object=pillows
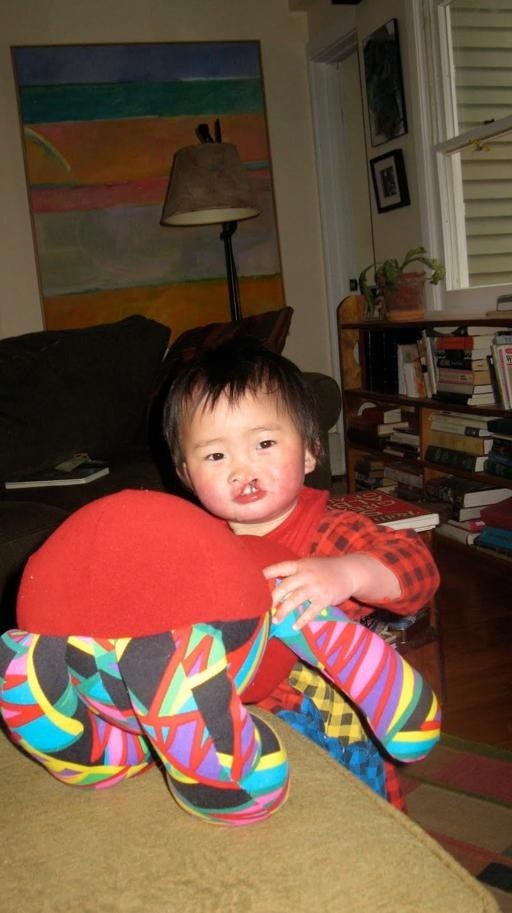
[143,306,293,442]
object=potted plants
[358,247,445,322]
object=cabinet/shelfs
[336,295,512,570]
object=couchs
[0,315,342,630]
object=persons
[161,333,442,819]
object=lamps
[163,119,260,321]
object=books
[3,465,111,490]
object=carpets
[399,735,510,913]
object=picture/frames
[362,17,411,214]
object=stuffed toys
[0,485,442,826]
